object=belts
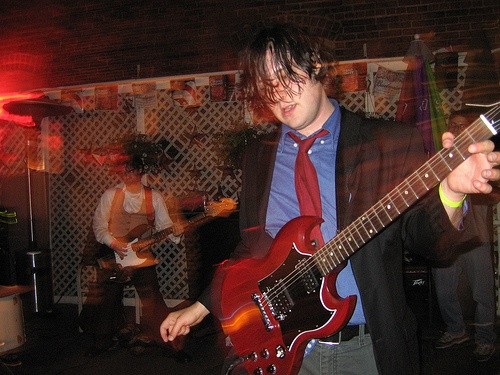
[315,323,369,345]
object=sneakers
[473,343,497,362]
[434,330,471,351]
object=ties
[288,129,329,251]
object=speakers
[403,264,436,324]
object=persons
[434,110,500,361]
[92,139,186,344]
[160,22,500,375]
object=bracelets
[439,182,467,208]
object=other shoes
[85,343,108,359]
[165,349,194,362]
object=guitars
[208,101,500,375]
[86,193,237,277]
